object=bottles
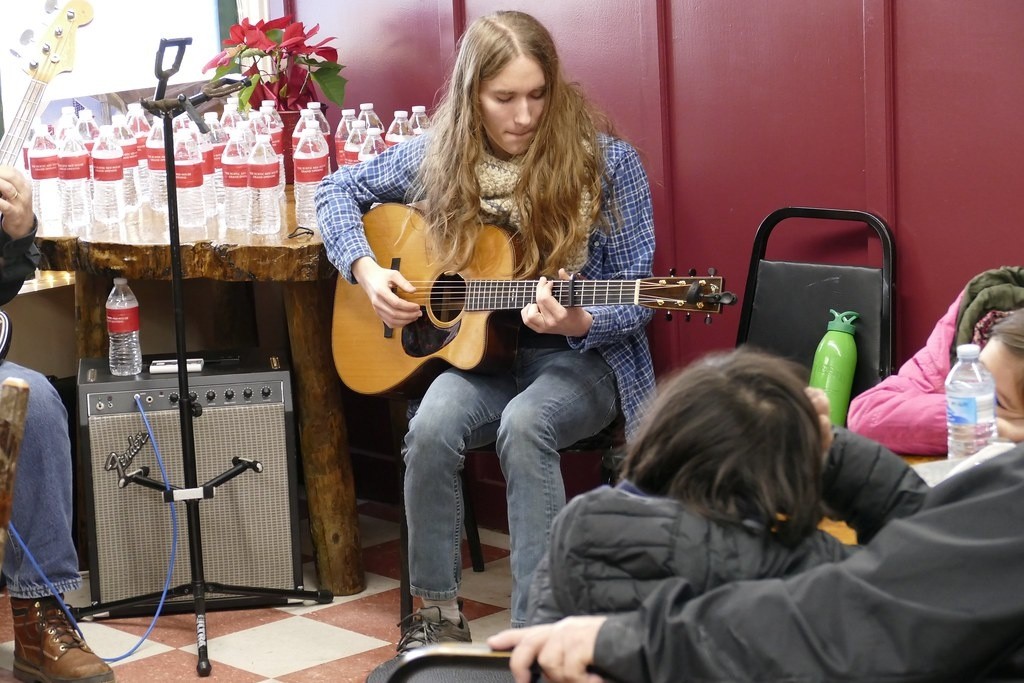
[945,344,997,474]
[19,98,434,236]
[105,278,142,377]
[809,309,860,428]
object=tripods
[63,37,336,674]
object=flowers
[200,14,349,109]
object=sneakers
[396,600,472,652]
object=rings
[8,193,18,200]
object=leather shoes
[10,593,114,683]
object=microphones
[231,456,264,473]
[119,466,149,489]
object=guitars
[324,192,746,401]
[0,0,101,374]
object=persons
[0,165,114,683]
[523,349,929,628]
[849,264,1023,454]
[315,11,654,656]
[488,444,1024,683]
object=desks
[22,183,369,596]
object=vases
[277,100,329,184]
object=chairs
[399,416,626,634]
[735,207,896,397]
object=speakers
[73,349,305,606]
